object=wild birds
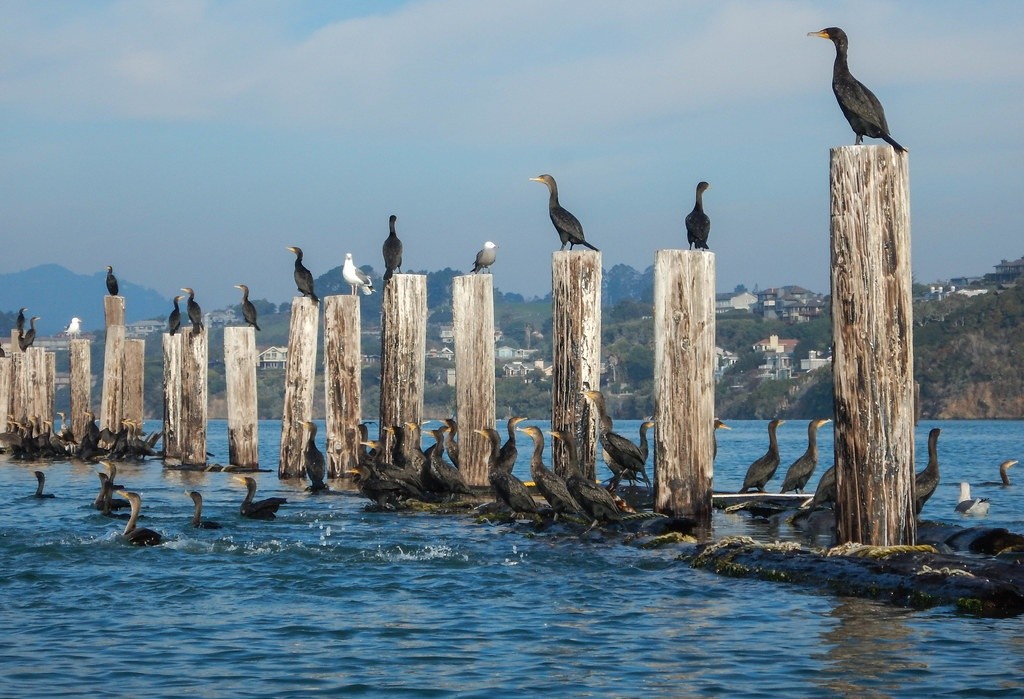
[180,288,204,330]
[33,471,54,498]
[529,175,598,251]
[106,265,118,296]
[299,418,475,511]
[685,182,711,250]
[382,215,403,280]
[475,392,654,538]
[65,317,81,340]
[914,427,1018,519]
[0,411,164,463]
[342,253,376,296]
[234,285,260,331]
[470,241,500,274]
[807,27,906,153]
[16,307,40,353]
[287,247,319,302]
[94,460,288,549]
[713,416,836,510]
[168,295,185,336]
